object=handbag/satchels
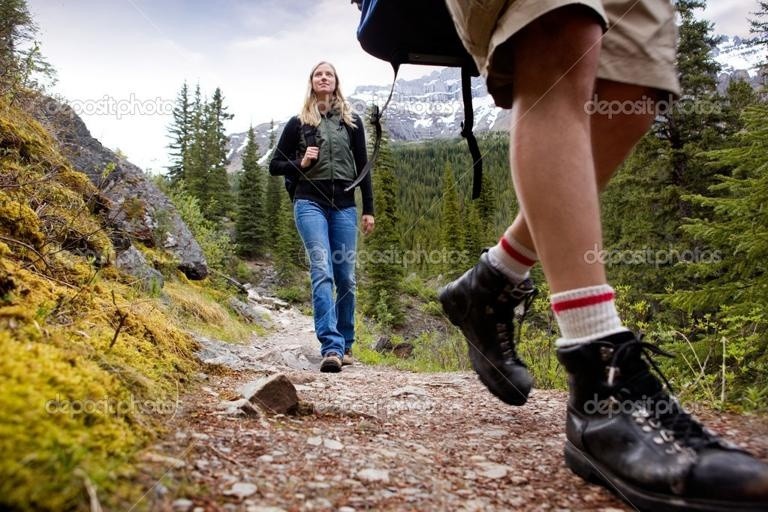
[285,114,316,202]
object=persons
[438,0,768,512]
[269,61,376,372]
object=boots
[557,331,768,512]
[438,252,531,405]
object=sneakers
[342,348,353,364]
[320,351,342,373]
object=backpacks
[351,0,480,76]
